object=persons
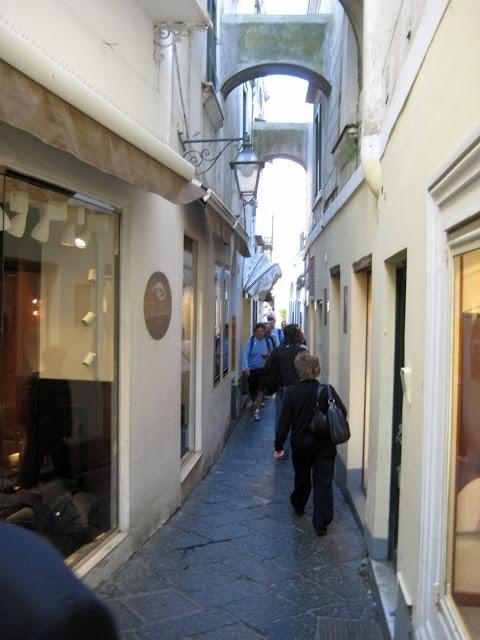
[272,352,348,537]
[216,324,229,374]
[21,346,75,488]
[181,326,191,426]
[242,316,310,460]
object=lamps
[179,130,265,204]
[0,190,97,369]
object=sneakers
[294,507,304,516]
[315,528,326,536]
[254,412,260,420]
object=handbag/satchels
[309,400,350,444]
[257,362,277,394]
